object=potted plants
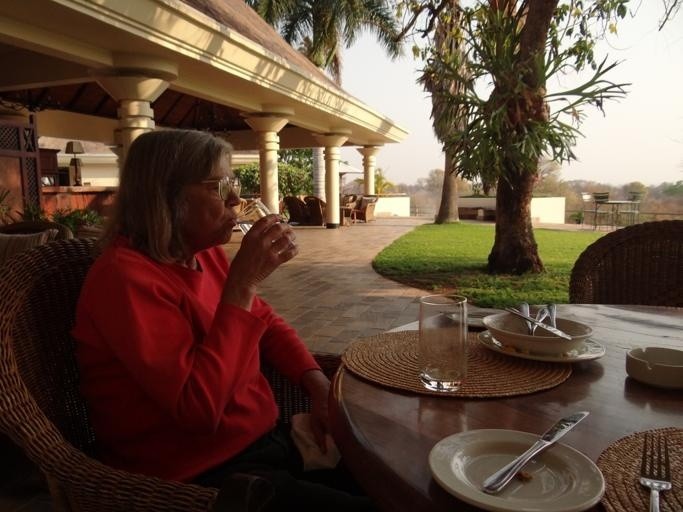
[625,184,641,200]
[571,205,584,224]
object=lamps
[66,141,84,187]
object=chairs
[569,220,683,304]
[1,238,341,509]
[283,194,379,226]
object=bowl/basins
[482,313,593,352]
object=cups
[419,294,469,392]
[233,197,271,234]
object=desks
[593,200,640,228]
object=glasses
[185,177,243,199]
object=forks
[639,433,673,511]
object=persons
[69,127,379,512]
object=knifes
[484,411,590,494]
[505,305,571,340]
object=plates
[442,308,493,329]
[428,428,606,511]
[477,330,606,364]
